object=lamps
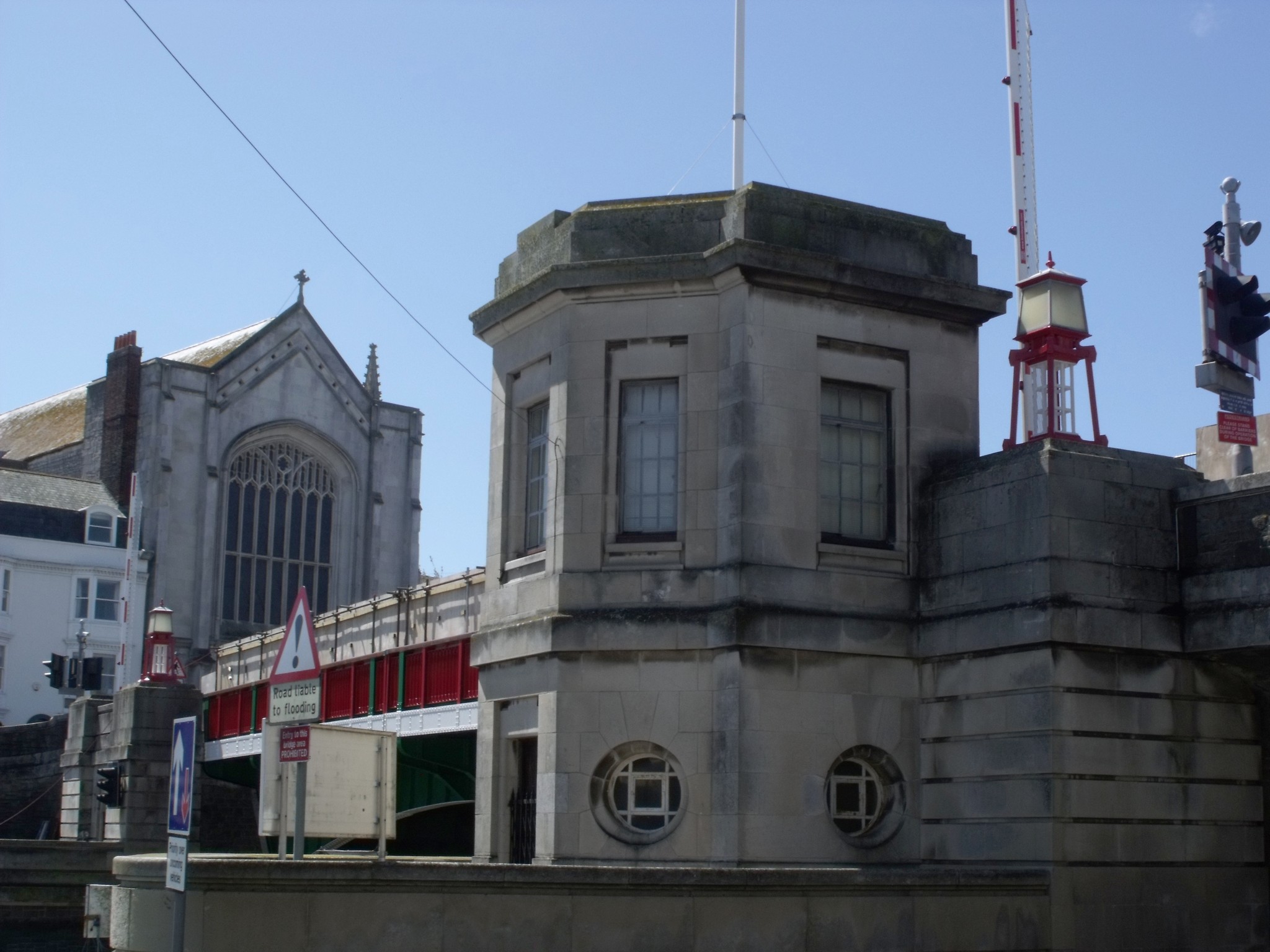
[1001,250,1109,451]
[139,598,178,684]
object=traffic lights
[41,649,61,689]
[83,656,103,691]
[1215,269,1268,358]
[93,763,124,810]
